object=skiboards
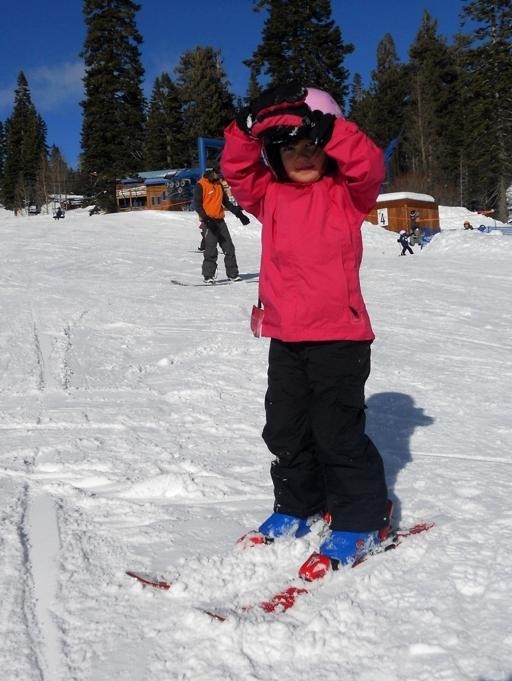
[125,508,435,622]
[172,274,260,287]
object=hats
[213,163,224,178]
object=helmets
[464,220,470,227]
[247,84,345,182]
[399,229,406,235]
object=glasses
[249,83,309,125]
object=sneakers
[297,498,394,582]
[202,276,215,283]
[235,509,323,551]
[228,275,242,281]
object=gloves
[305,106,336,149]
[202,215,221,235]
[235,98,261,140]
[237,211,250,225]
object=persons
[194,163,252,284]
[463,221,474,230]
[409,208,424,249]
[55,207,63,221]
[218,86,393,563]
[396,230,415,256]
